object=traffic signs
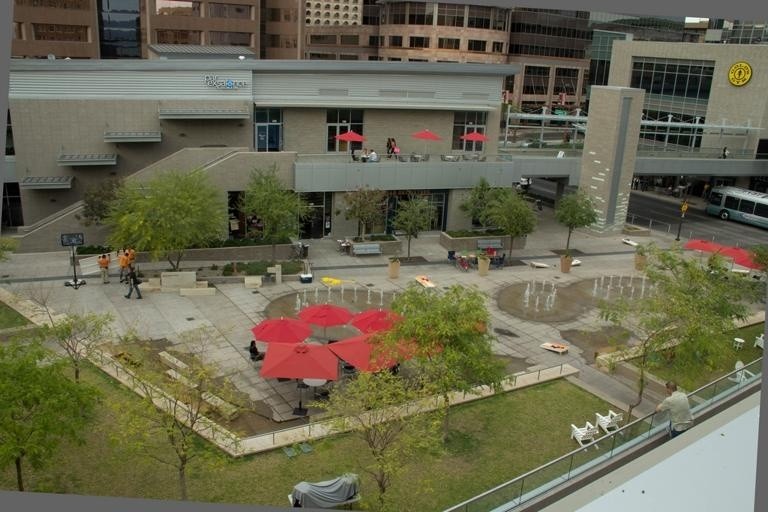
[553,109,566,115]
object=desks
[335,238,351,256]
[286,491,364,512]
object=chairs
[293,363,355,401]
[594,407,627,438]
[246,348,264,369]
[350,151,488,164]
[446,245,507,276]
[569,420,599,452]
[734,360,755,383]
[700,262,762,282]
[753,331,765,350]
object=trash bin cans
[301,243,309,258]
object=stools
[733,336,746,351]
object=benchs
[476,238,505,252]
[352,242,383,258]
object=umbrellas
[734,254,761,272]
[410,130,442,153]
[335,130,366,155]
[682,239,721,263]
[717,247,749,267]
[460,133,490,141]
[251,304,444,411]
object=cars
[570,122,586,133]
[518,177,532,186]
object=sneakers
[124,294,131,299]
[135,296,144,300]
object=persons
[98,246,143,299]
[360,148,369,162]
[369,149,378,162]
[656,380,694,437]
[249,340,265,361]
[723,146,727,158]
[632,176,639,190]
[386,138,392,154]
[388,138,397,159]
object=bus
[702,184,767,230]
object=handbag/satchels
[135,279,142,285]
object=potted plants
[386,251,401,278]
[558,252,574,274]
[633,243,648,272]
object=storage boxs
[299,273,314,283]
